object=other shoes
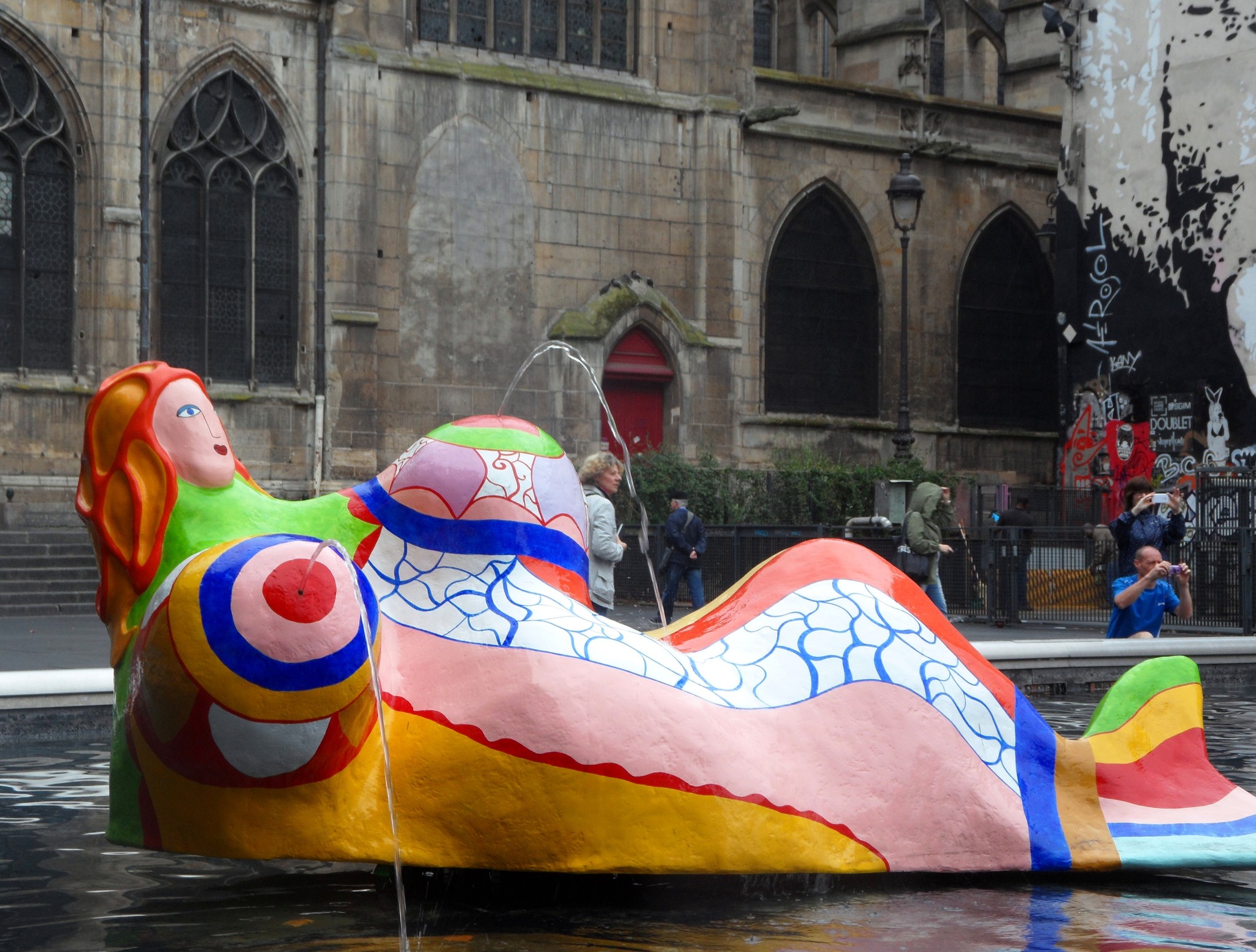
[1019,604,1033,610]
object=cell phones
[1151,494,1171,504]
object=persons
[1106,545,1194,642]
[995,495,1036,612]
[1108,477,1187,579]
[647,488,708,627]
[65,354,1254,884]
[969,508,1002,573]
[573,450,629,617]
[895,480,957,621]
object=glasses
[1134,491,1151,496]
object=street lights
[1036,215,1069,489]
[885,152,928,467]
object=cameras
[1166,565,1181,574]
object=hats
[671,491,688,499]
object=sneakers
[649,617,669,626]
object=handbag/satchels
[892,542,931,579]
[657,545,674,573]
